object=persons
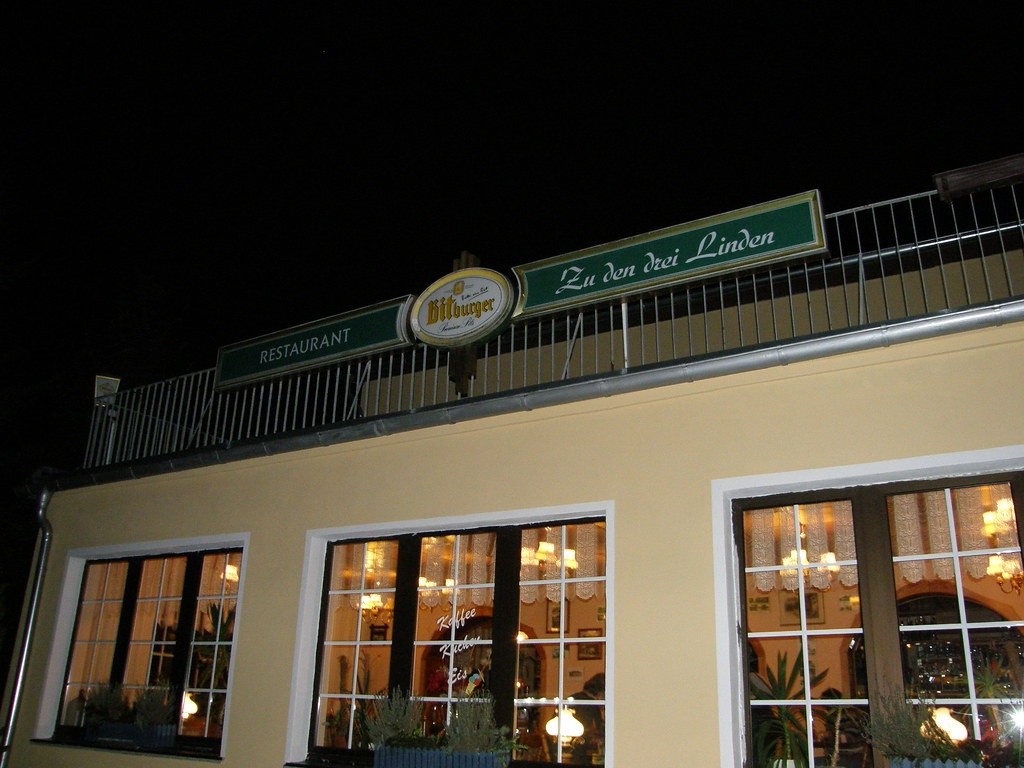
[820,688,869,751]
[548,672,606,765]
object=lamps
[219,565,239,594]
[418,577,458,613]
[357,594,395,624]
[780,521,839,594]
[981,498,1024,595]
[546,708,585,763]
[521,541,579,578]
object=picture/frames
[577,628,603,660]
[778,587,826,627]
[546,597,570,634]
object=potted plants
[84,678,182,748]
[364,685,528,768]
[861,681,982,768]
[748,646,829,768]
